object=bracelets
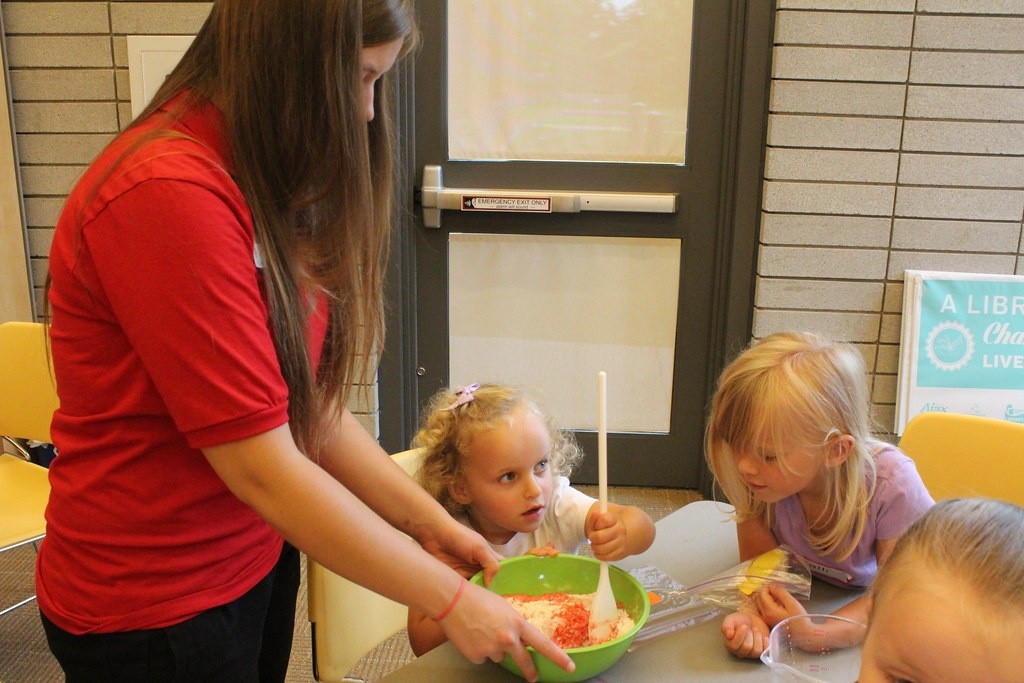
[430,576,468,621]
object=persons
[406,384,657,657]
[859,499,1024,683]
[36,0,577,683]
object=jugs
[760,613,869,683]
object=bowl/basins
[467,552,650,683]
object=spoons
[585,371,620,644]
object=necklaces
[704,330,935,659]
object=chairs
[898,412,1024,512]
[308,447,430,683]
[0,320,62,617]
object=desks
[374,501,866,683]
